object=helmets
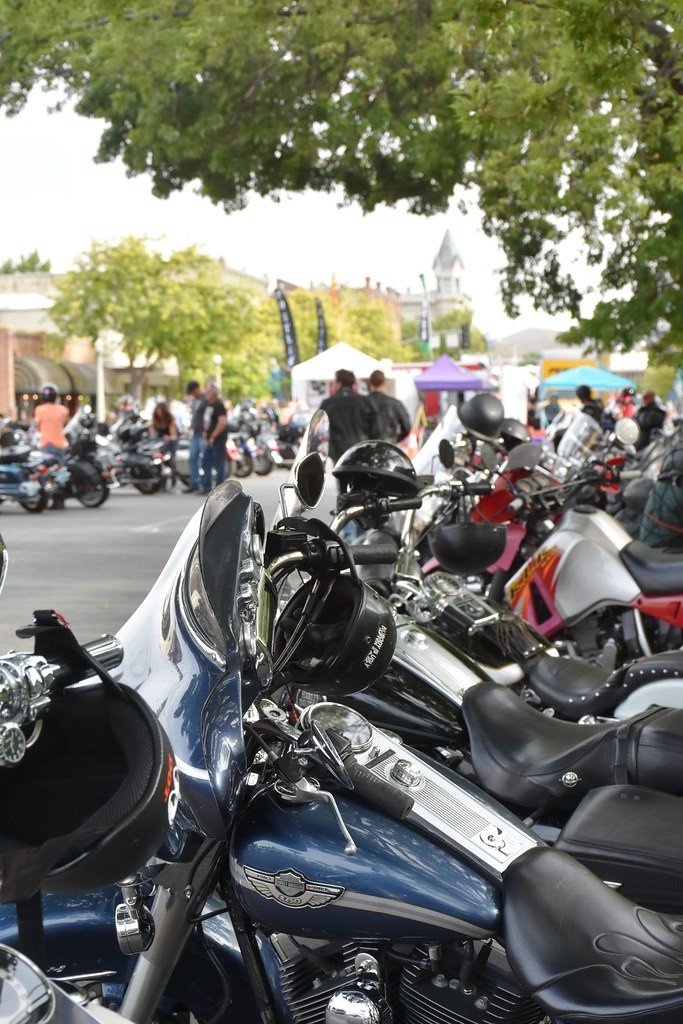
[332,439,418,499]
[272,571,397,697]
[427,523,507,576]
[457,394,505,442]
[614,417,640,445]
[502,418,531,443]
[0,646,183,906]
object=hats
[120,395,134,406]
[186,382,199,393]
[42,387,57,401]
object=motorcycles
[0,397,683,1023]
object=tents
[464,351,683,428]
[291,342,420,435]
[414,353,500,391]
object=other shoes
[179,476,191,487]
[181,486,199,494]
[50,502,64,511]
[197,489,212,497]
[171,478,177,489]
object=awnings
[14,356,72,395]
[61,362,113,396]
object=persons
[367,370,411,446]
[617,388,636,419]
[576,386,602,426]
[546,395,560,423]
[33,387,70,509]
[184,381,212,492]
[635,392,666,451]
[320,369,374,494]
[148,403,180,486]
[198,386,229,495]
[108,394,139,422]
